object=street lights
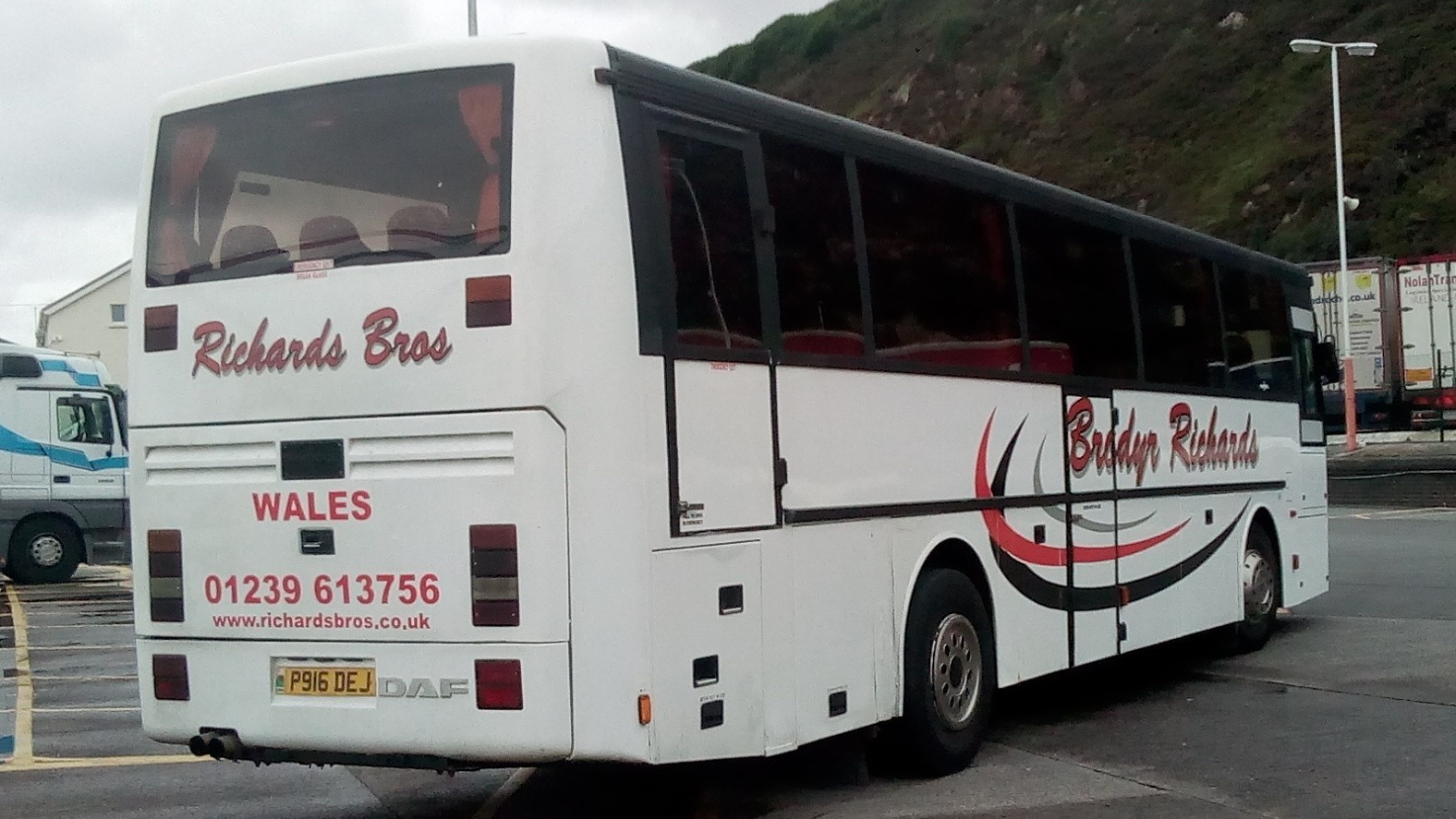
[1288,39,1378,454]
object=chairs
[217,207,449,268]
[677,329,1069,380]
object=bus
[121,34,1329,782]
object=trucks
[0,341,131,587]
